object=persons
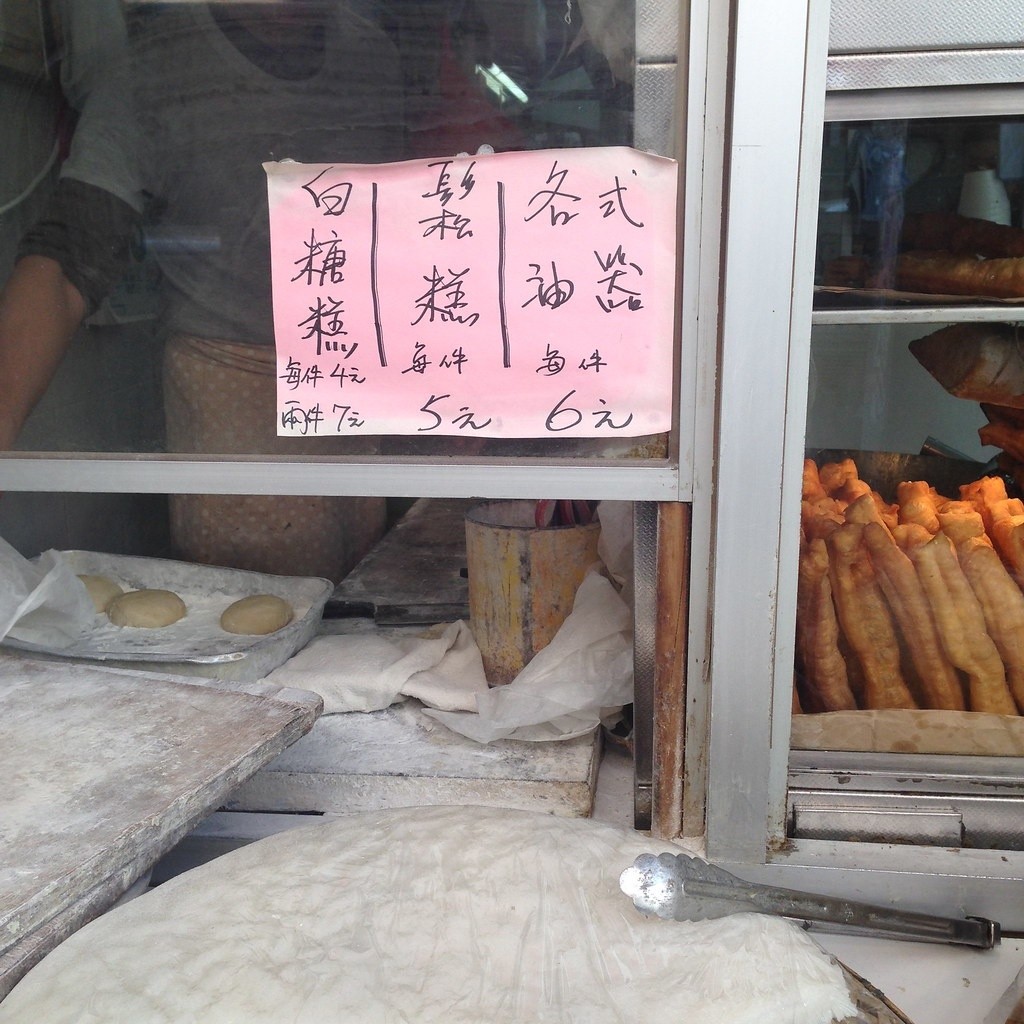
[0,0,633,608]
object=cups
[462,500,601,685]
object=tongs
[621,852,1000,951]
